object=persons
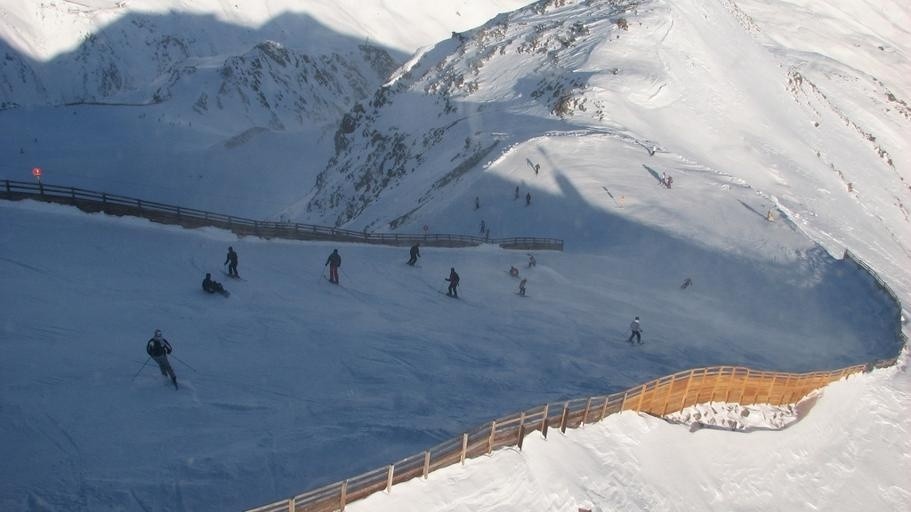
[660,172,666,184]
[445,267,460,298]
[325,248,341,284]
[475,197,480,208]
[528,256,533,267]
[480,220,486,233]
[629,316,643,344]
[202,272,230,298]
[223,246,238,277]
[146,329,177,385]
[667,177,673,188]
[680,278,693,290]
[510,266,519,276]
[526,192,531,205]
[407,242,421,264]
[515,186,519,198]
[535,164,540,175]
[618,196,624,208]
[651,145,656,155]
[519,278,527,295]
[485,228,490,239]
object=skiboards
[323,275,346,290]
[161,373,179,392]
[432,288,463,300]
[219,268,249,282]
